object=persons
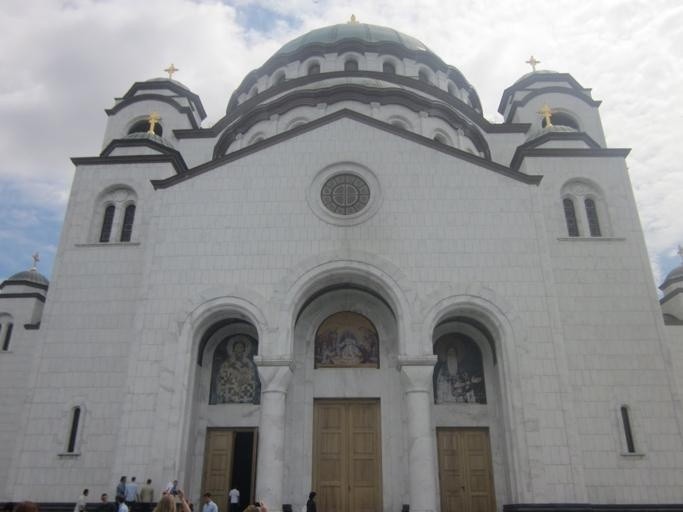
[95,473,268,512]
[306,492,318,511]
[73,489,89,512]
[314,326,380,369]
[216,336,258,405]
[434,344,482,404]
